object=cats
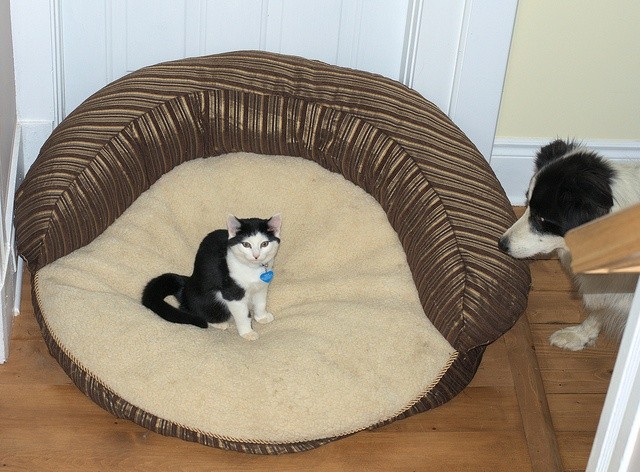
[139,213,282,341]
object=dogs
[498,134,639,352]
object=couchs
[13,48,531,456]
